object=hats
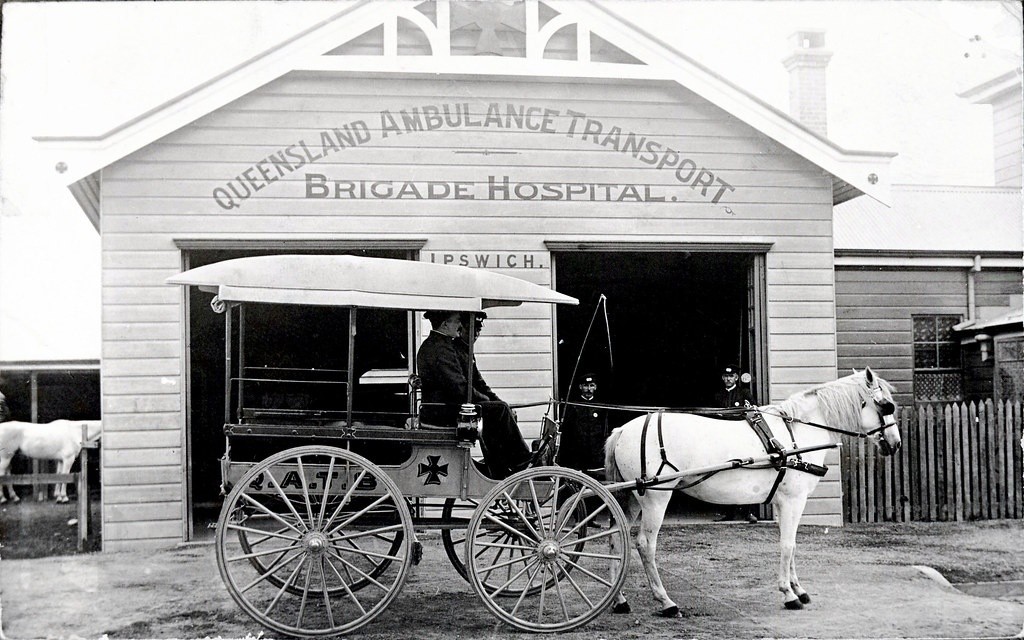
[424,311,457,319]
[461,312,487,328]
[579,374,599,383]
[721,364,741,374]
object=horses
[0,419,102,504]
[605,365,901,620]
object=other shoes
[714,512,760,523]
[515,451,538,466]
[573,517,601,529]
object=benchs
[408,378,458,432]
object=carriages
[165,255,903,640]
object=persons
[569,374,606,528]
[416,309,545,480]
[712,366,764,522]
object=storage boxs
[359,368,413,429]
